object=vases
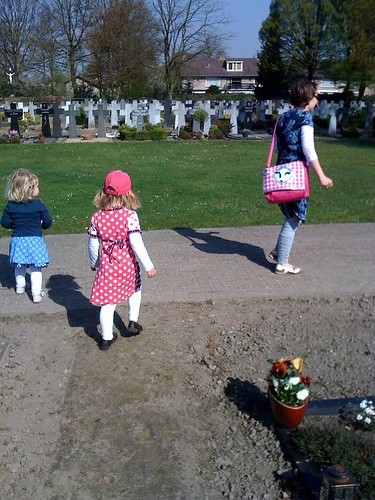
[267,384,310,429]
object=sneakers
[266,251,278,264]
[275,263,300,275]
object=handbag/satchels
[262,161,310,203]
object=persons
[0,168,53,304]
[87,170,157,350]
[268,79,333,274]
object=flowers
[265,357,312,408]
[356,399,375,430]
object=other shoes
[128,321,140,333]
[16,282,31,294]
[33,292,46,303]
[100,333,117,350]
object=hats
[104,170,131,196]
